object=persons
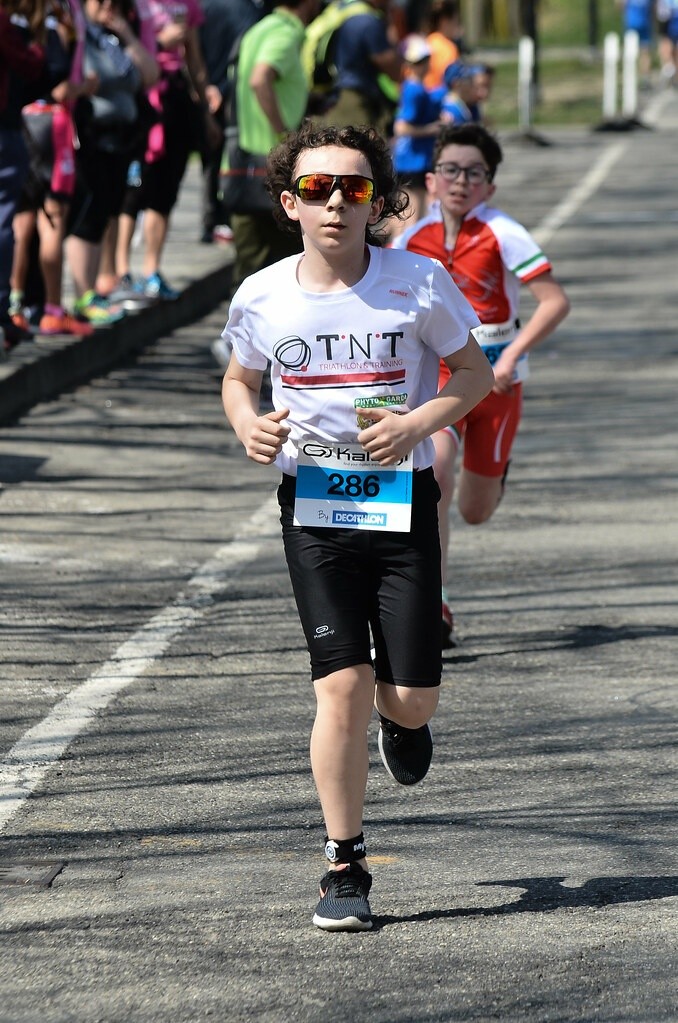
[613,0,678,91]
[0,0,258,358]
[222,128,494,930]
[211,0,493,368]
[389,124,569,645]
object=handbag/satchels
[223,150,279,213]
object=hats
[399,33,431,64]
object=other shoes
[210,336,272,388]
[496,463,511,505]
[441,602,453,631]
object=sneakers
[73,272,178,325]
[2,313,30,351]
[40,303,96,337]
[377,711,434,787]
[312,861,373,929]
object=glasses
[289,174,378,204]
[434,162,491,185]
